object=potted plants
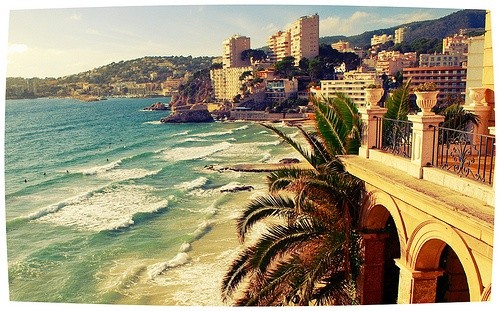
[413,82,439,116]
[363,84,384,109]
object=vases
[467,87,486,106]
